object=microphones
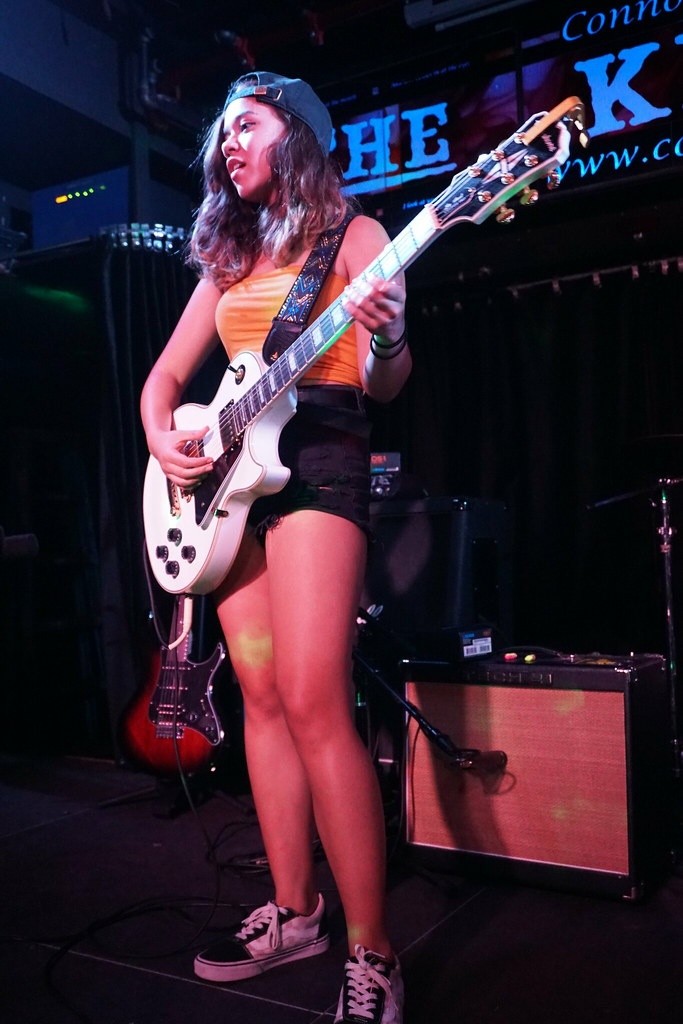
[448,751,506,775]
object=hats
[223,71,333,164]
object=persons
[141,71,412,1024]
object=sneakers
[331,943,404,1024]
[193,893,333,981]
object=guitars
[138,92,596,598]
[117,594,227,780]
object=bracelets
[369,329,406,360]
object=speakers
[349,494,511,796]
[396,654,678,889]
